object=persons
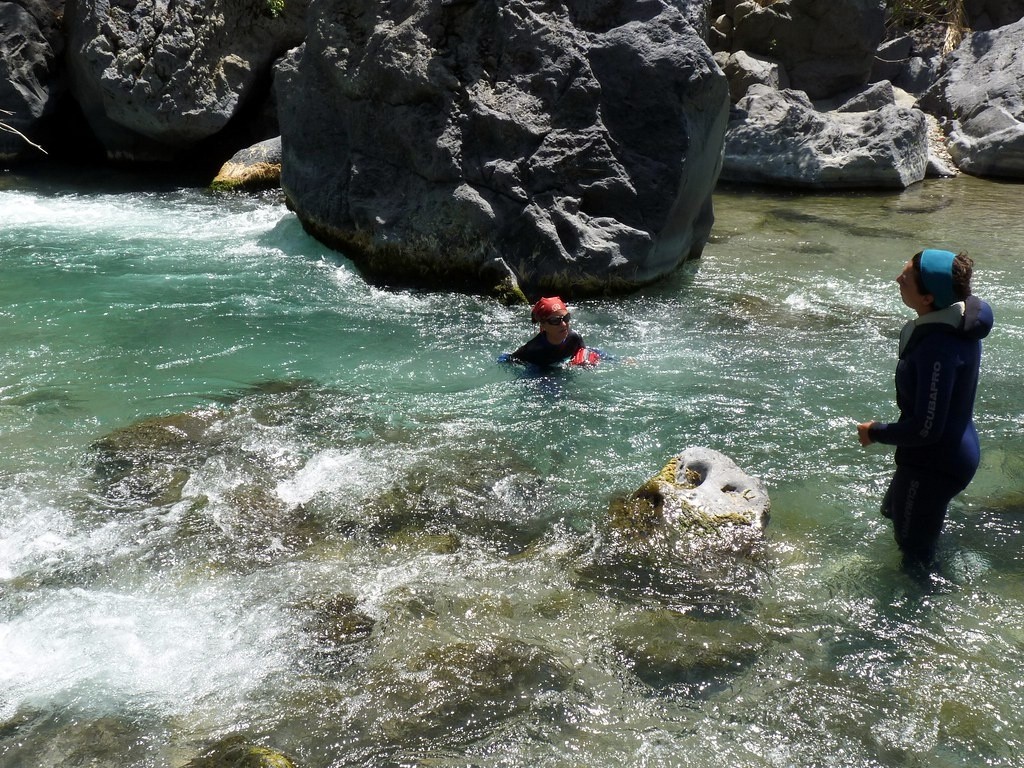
[857,247,993,550]
[512,296,584,361]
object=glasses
[545,313,570,326]
